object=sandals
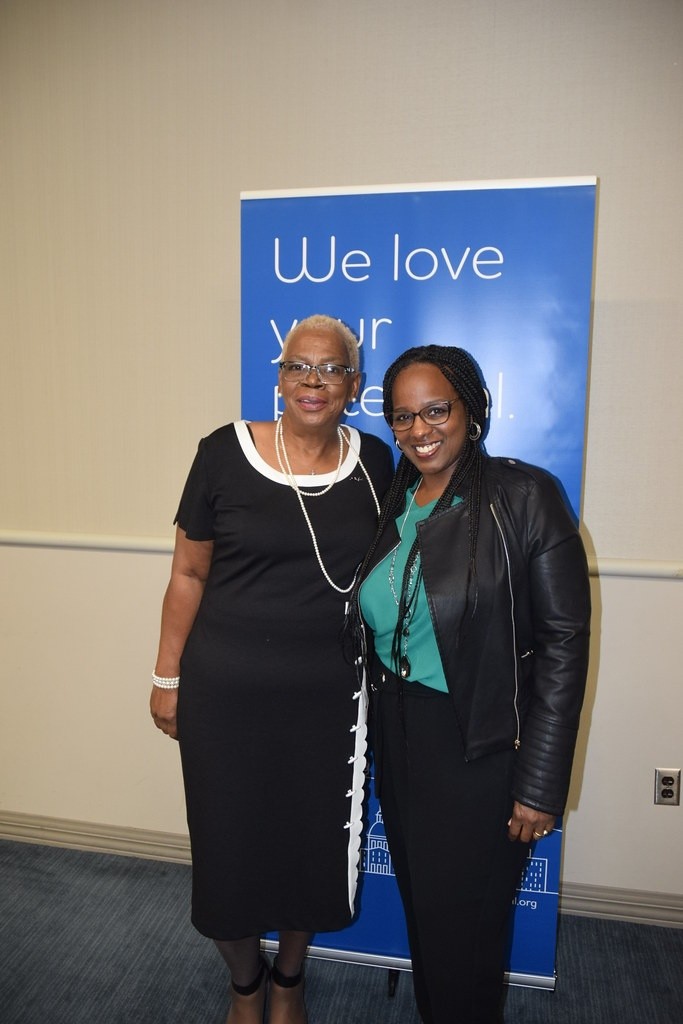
[272,959,308,1024]
[228,963,271,1024]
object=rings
[533,830,548,838]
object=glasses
[279,359,354,385]
[385,397,459,433]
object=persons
[338,344,592,1024]
[149,313,396,1024]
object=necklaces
[151,670,180,689]
[388,477,423,678]
[275,415,381,593]
